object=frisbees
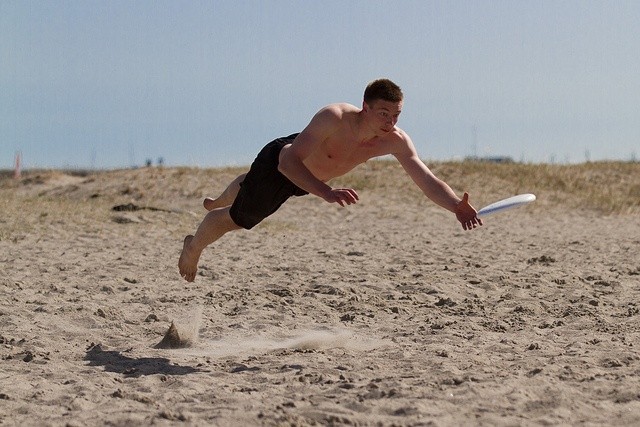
[477,193,536,214]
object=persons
[177,78,482,283]
